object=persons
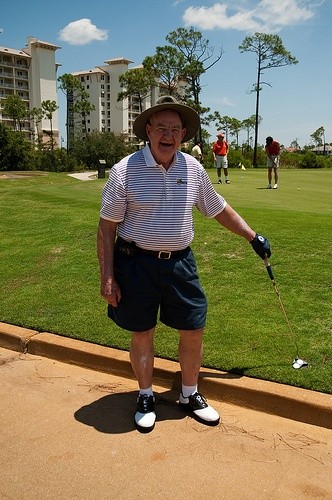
[190,141,202,165]
[264,134,281,189]
[96,96,274,432]
[211,132,231,185]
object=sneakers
[178,392,220,426]
[134,394,156,432]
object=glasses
[221,137,224,139]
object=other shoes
[267,184,271,188]
[218,179,221,184]
[273,184,278,189]
[226,180,230,184]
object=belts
[116,237,190,260]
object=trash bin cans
[98,160,106,178]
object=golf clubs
[264,258,308,369]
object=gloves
[249,233,271,259]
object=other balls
[298,188,301,191]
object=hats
[133,96,200,144]
[217,133,225,138]
